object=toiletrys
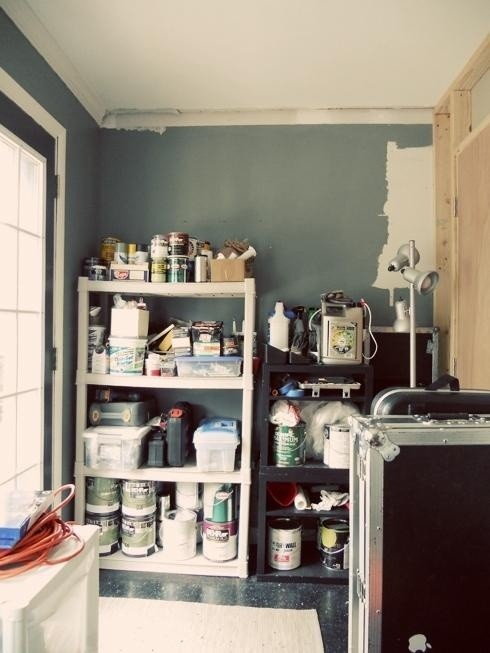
[294,485,307,511]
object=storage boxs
[212,258,251,282]
[175,356,243,377]
[193,417,240,472]
[82,426,152,472]
[89,401,148,426]
[110,306,148,338]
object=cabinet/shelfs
[73,278,256,580]
[255,322,440,584]
[0,523,102,653]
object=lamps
[388,238,439,388]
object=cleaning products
[267,301,290,353]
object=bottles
[267,299,322,355]
[194,239,213,281]
[114,242,149,264]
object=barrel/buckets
[161,509,197,560]
[267,517,302,571]
[273,425,308,468]
[202,519,238,562]
[318,518,349,571]
[108,337,147,375]
[85,480,157,556]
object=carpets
[98,594,325,653]
